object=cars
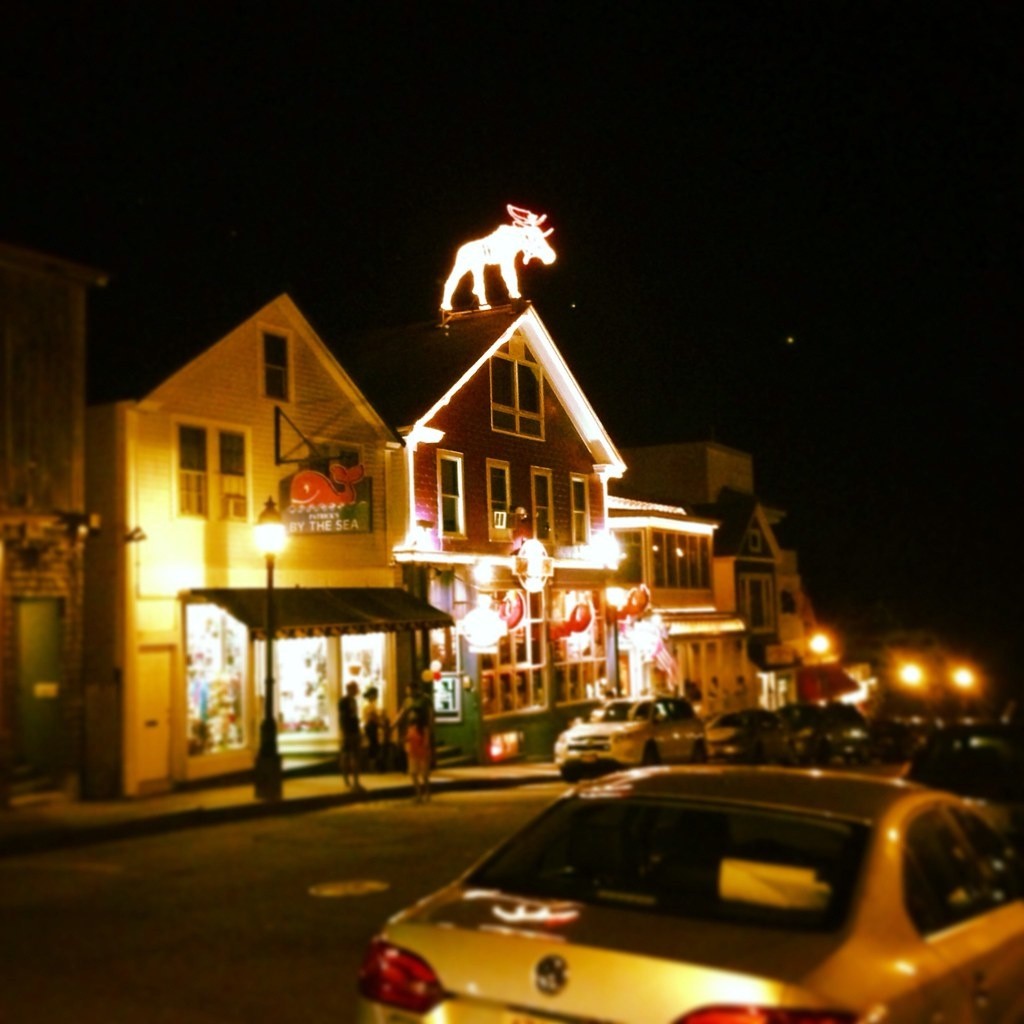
[353,764,1023,1023]
[900,723,1023,800]
[706,703,870,767]
[553,694,704,782]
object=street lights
[253,497,282,798]
[606,576,625,696]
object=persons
[389,685,432,733]
[336,682,367,792]
[362,689,386,774]
[403,706,433,805]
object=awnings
[199,585,454,637]
